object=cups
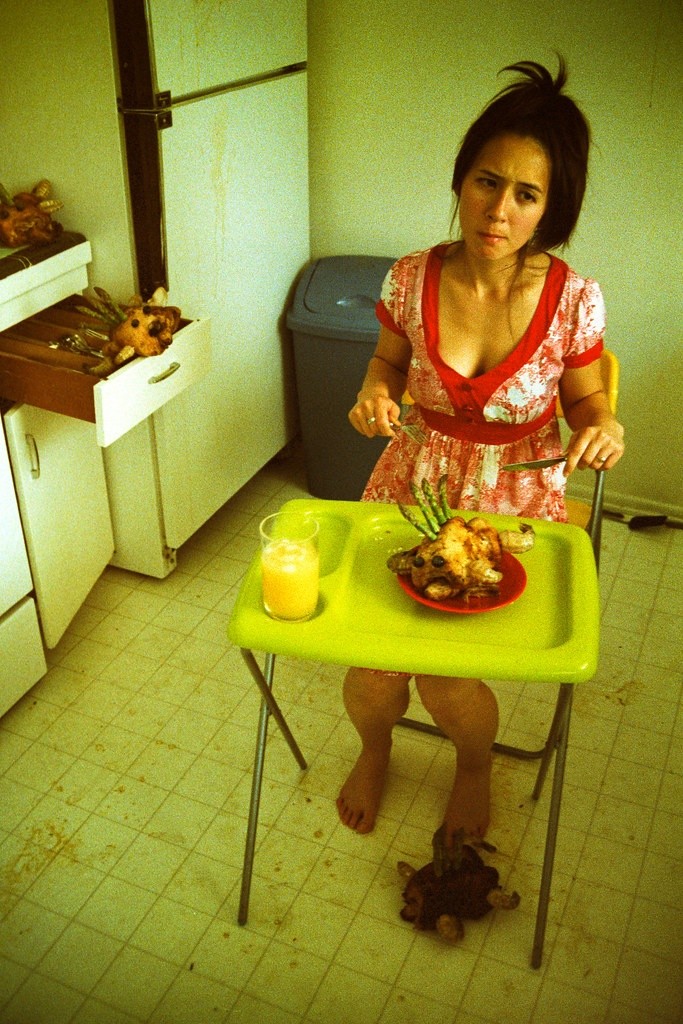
[258,510,323,624]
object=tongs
[74,286,128,327]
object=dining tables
[224,495,603,969]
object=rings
[597,455,606,463]
[366,418,375,424]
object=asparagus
[75,286,128,332]
[397,473,454,541]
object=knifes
[500,455,570,473]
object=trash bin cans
[286,256,401,503]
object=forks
[47,327,114,358]
[390,421,432,448]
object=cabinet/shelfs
[1,231,214,722]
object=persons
[331,48,627,858]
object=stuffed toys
[387,842,522,948]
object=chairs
[390,348,621,761]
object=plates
[397,541,529,616]
